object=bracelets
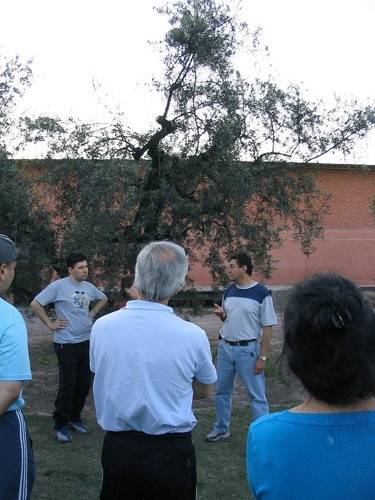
[92,308,96,313]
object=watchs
[258,355,267,361]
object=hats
[0,234,29,264]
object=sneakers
[53,424,73,443]
[205,430,230,441]
[67,420,89,434]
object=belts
[220,336,257,346]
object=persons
[246,272,375,500]
[113,276,138,311]
[206,253,276,441]
[30,253,108,442]
[0,234,36,500]
[89,241,219,500]
